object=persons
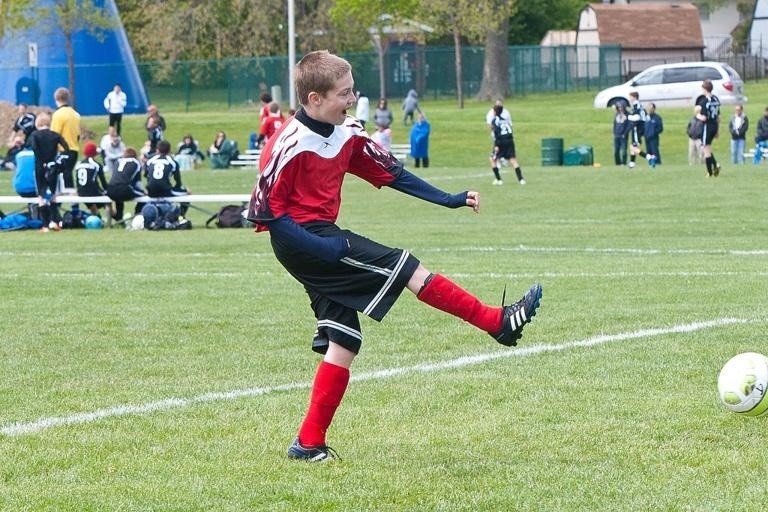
[100,126,125,170]
[146,140,191,219]
[207,130,233,169]
[255,93,296,149]
[486,99,517,168]
[612,75,768,177]
[2,88,82,233]
[146,105,167,157]
[409,112,430,168]
[108,148,149,224]
[103,84,127,136]
[356,88,423,152]
[488,105,527,186]
[247,53,543,467]
[175,136,197,158]
[75,141,116,219]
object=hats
[83,142,96,157]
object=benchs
[131,191,262,230]
[229,142,412,173]
[1,193,113,228]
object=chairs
[210,141,233,169]
[174,140,202,167]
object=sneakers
[488,283,542,346]
[491,161,528,186]
[631,153,721,177]
[287,436,327,462]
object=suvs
[594,59,747,109]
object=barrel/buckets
[540,136,564,167]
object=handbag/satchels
[217,205,242,227]
[143,199,192,230]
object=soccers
[717,352,768,418]
